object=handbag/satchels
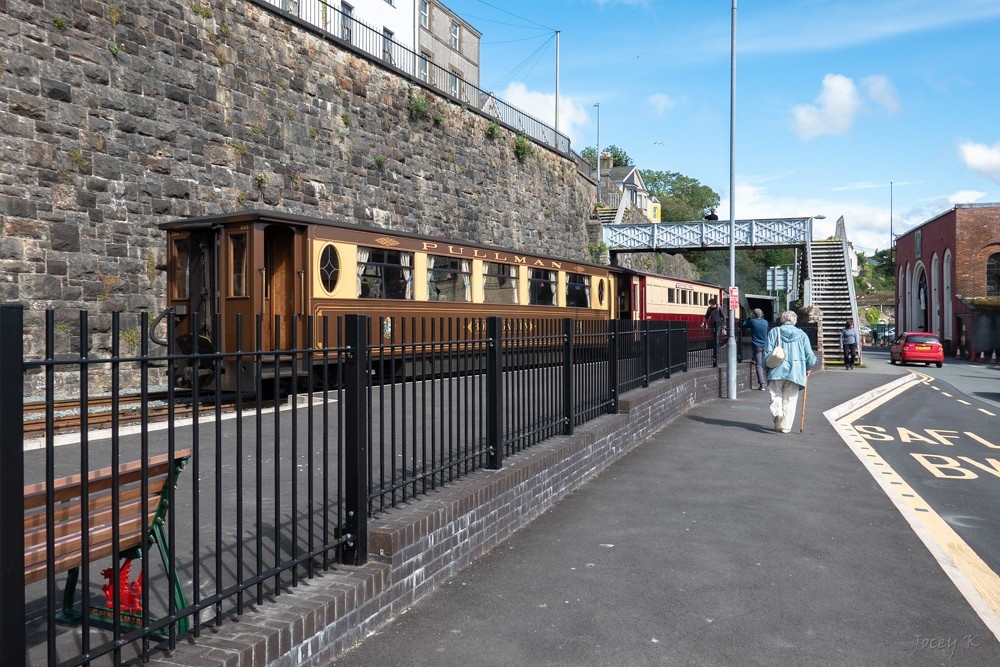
[766,346,787,368]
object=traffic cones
[862,337,866,346]
[871,338,875,347]
[990,348,999,364]
[955,346,962,359]
[979,349,986,363]
[879,336,884,348]
[969,351,976,362]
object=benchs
[24,448,193,641]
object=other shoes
[759,383,765,390]
[850,364,854,370]
[846,366,850,370]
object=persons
[700,298,727,358]
[840,321,858,370]
[743,308,769,391]
[704,210,719,220]
[761,311,817,434]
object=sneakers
[775,415,783,432]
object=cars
[891,331,944,368]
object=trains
[155,209,748,393]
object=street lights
[807,214,826,307]
[593,102,601,183]
